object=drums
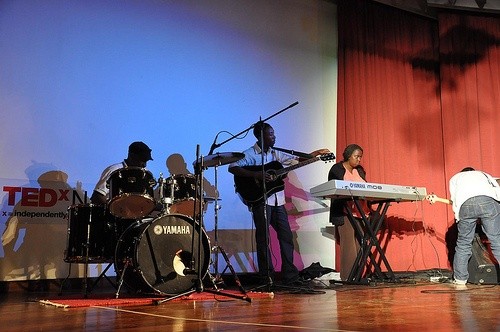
[114,214,212,297]
[107,168,156,220]
[161,172,206,218]
[62,202,115,264]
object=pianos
[310,179,427,287]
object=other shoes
[452,278,467,284]
[291,280,306,285]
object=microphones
[208,136,216,155]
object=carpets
[26,287,275,309]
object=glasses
[351,154,362,159]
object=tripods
[156,143,252,305]
[216,101,325,295]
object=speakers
[466,233,498,284]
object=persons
[328,144,370,281]
[449,167,500,285]
[228,123,330,285]
[92,140,153,204]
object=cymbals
[204,195,221,202]
[192,152,246,168]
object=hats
[130,141,153,161]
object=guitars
[234,153,336,206]
[426,191,453,206]
